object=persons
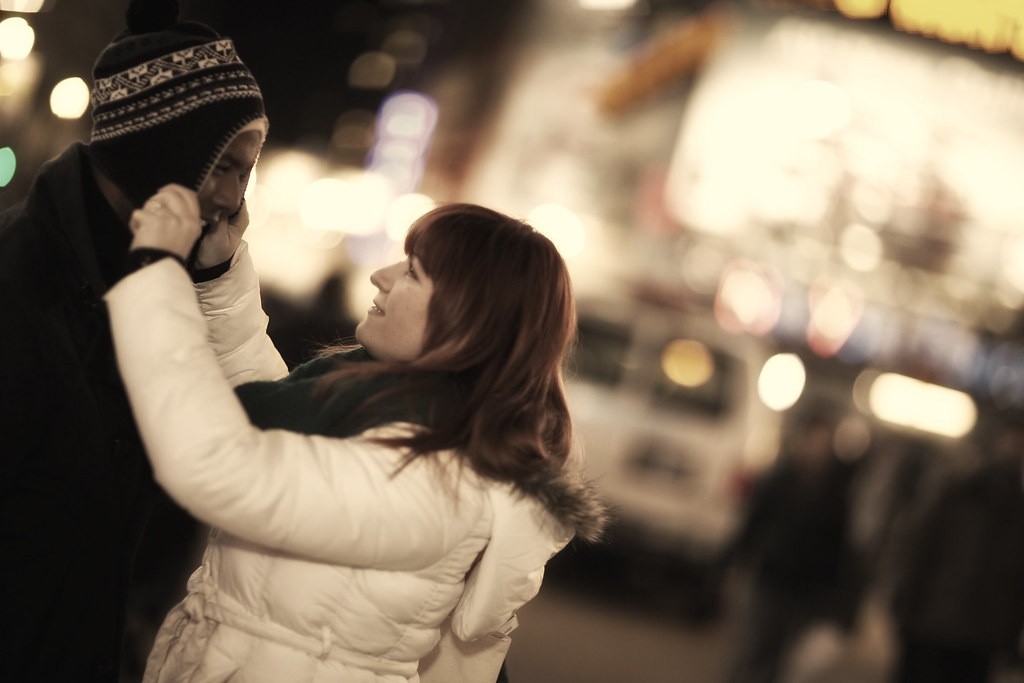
[714,408,1024,683]
[0,19,270,683]
[101,185,611,683]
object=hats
[89,0,270,229]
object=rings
[153,200,163,209]
